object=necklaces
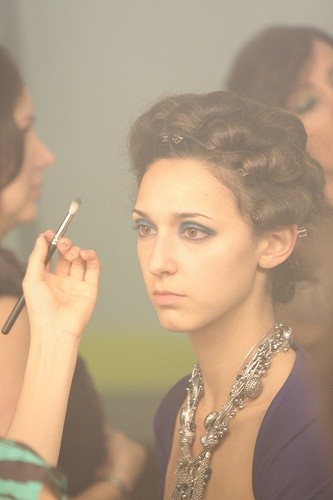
[169,321,294,500]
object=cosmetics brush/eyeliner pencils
[2,196,82,336]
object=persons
[128,92,333,500]
[0,230,98,500]
[225,27,333,368]
[0,46,149,498]
